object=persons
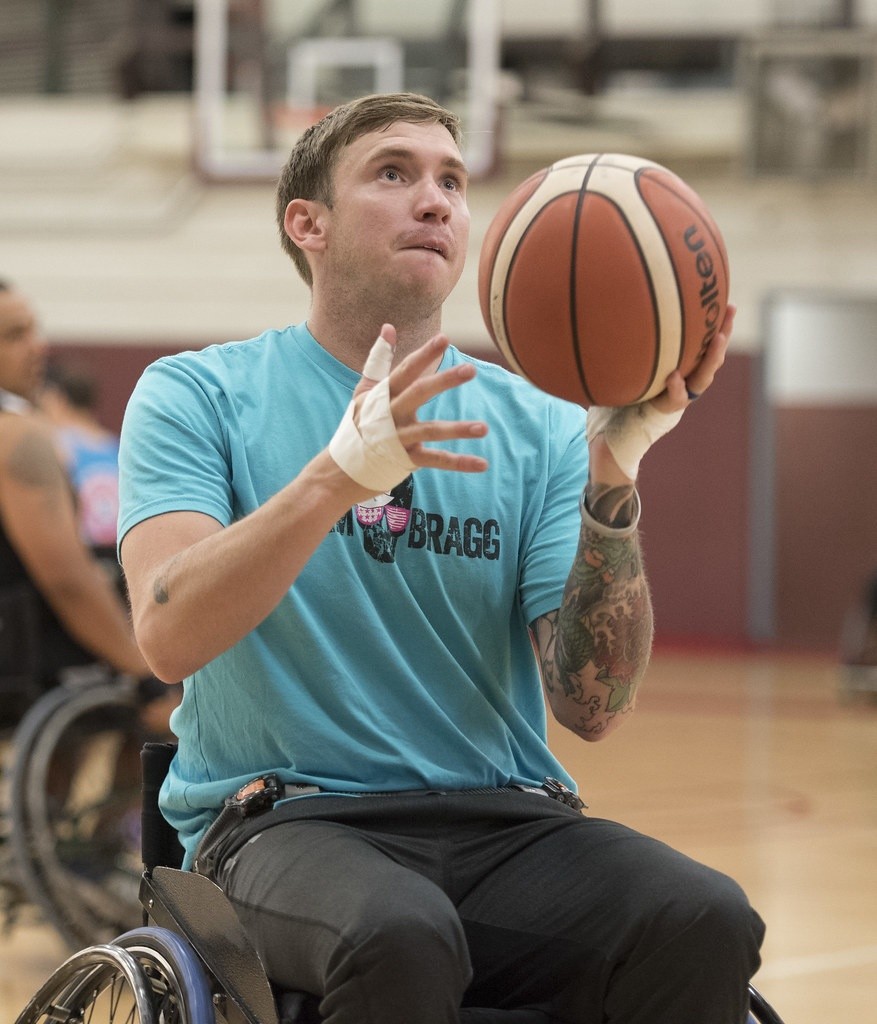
[31,357,119,555]
[115,94,763,1023]
[0,280,184,857]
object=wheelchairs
[0,669,783,1024]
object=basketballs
[474,152,731,413]
